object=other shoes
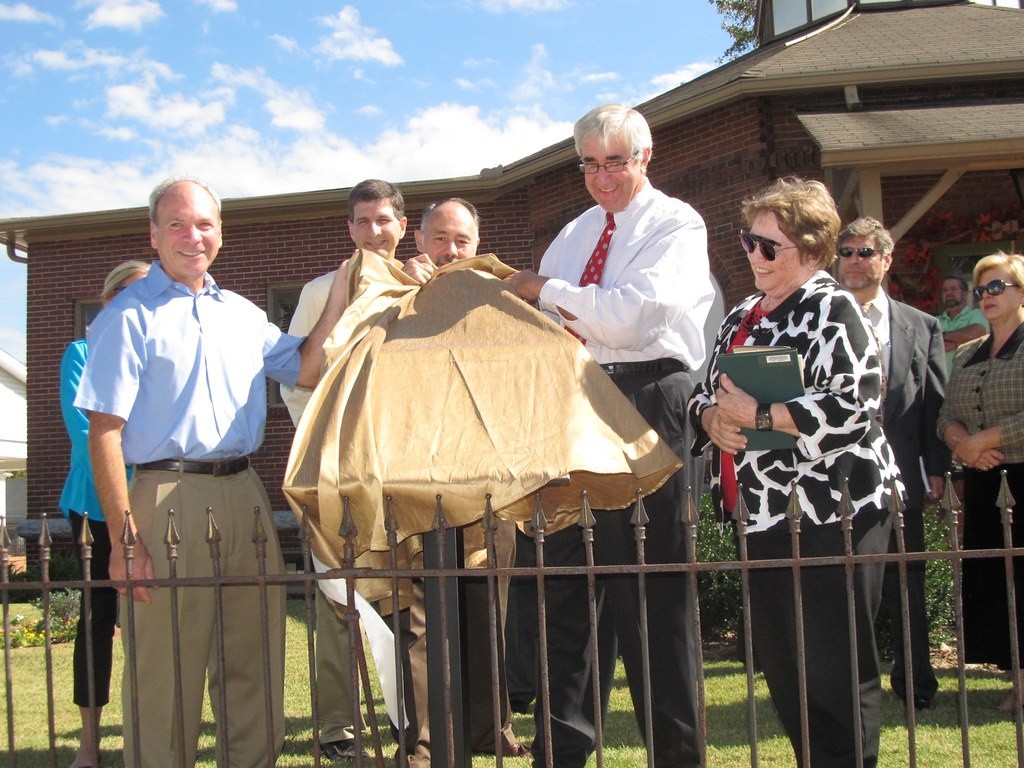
[322,739,369,762]
[487,739,531,757]
[902,689,932,710]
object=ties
[565,212,615,345]
[861,303,887,426]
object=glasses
[575,151,639,174]
[839,247,883,257]
[740,234,799,261]
[972,280,1020,301]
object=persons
[935,252,1024,711]
[58,260,151,768]
[928,276,990,548]
[835,217,952,708]
[278,180,419,763]
[393,197,531,768]
[689,175,910,768]
[74,175,359,768]
[501,106,716,768]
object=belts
[137,458,248,476]
[598,358,684,375]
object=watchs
[755,400,772,432]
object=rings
[989,465,991,468]
[984,466,985,470]
